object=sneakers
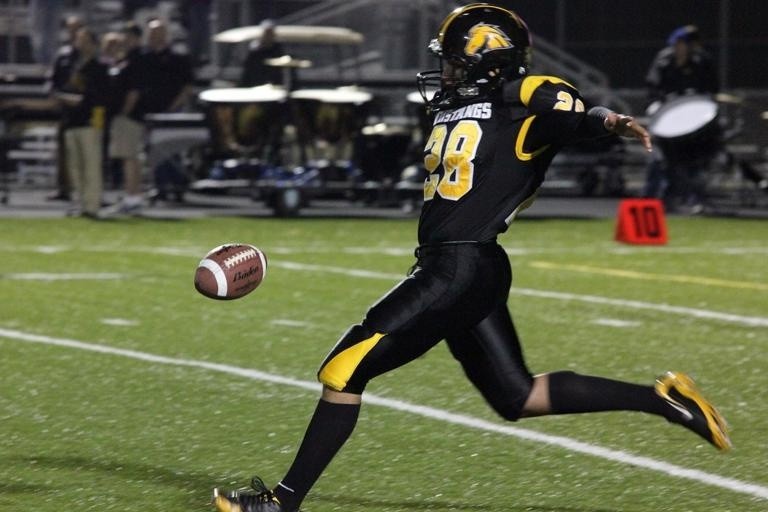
[653,370,732,452]
[214,475,301,511]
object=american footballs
[194,244,267,300]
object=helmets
[416,2,532,116]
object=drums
[199,86,283,167]
[362,125,410,170]
[649,93,720,161]
[289,87,370,166]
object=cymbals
[265,56,308,65]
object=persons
[238,17,298,90]
[210,1,732,512]
[644,24,768,199]
[46,14,191,220]
